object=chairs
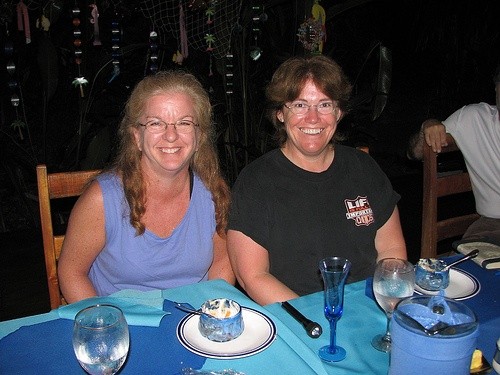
[422,131,478,261]
[35,164,106,311]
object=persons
[404,79,500,258]
[225,55,408,306]
[58,69,235,304]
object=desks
[266,243,499,375]
[0,278,315,375]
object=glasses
[284,102,340,116]
[138,119,199,134]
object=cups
[414,258,449,291]
[199,297,244,341]
[71,304,130,375]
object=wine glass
[318,256,352,362]
[371,258,414,353]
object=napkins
[56,288,171,328]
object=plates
[414,265,481,302]
[176,304,277,360]
[468,346,491,375]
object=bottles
[386,294,479,375]
[492,338,500,375]
